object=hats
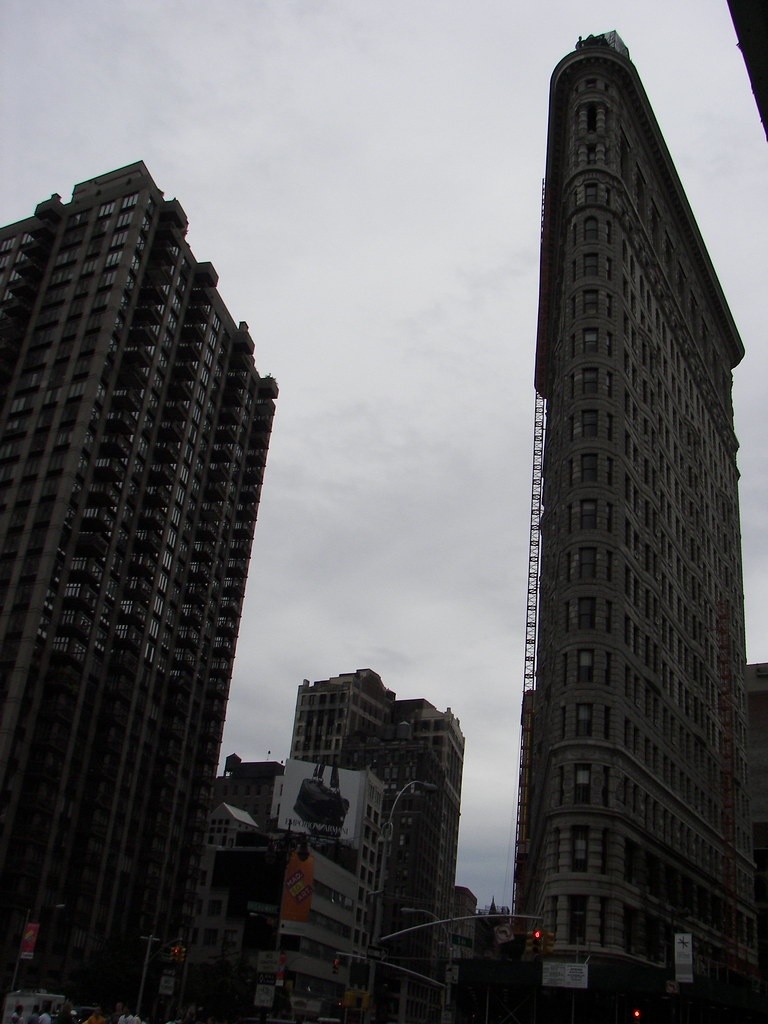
[16,1005,23,1011]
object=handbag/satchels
[293,761,349,838]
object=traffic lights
[532,928,543,954]
[174,945,180,962]
[337,1001,342,1007]
[170,946,176,961]
[332,959,339,974]
[180,947,186,963]
[631,1008,642,1023]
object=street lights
[686,1001,694,1024]
[361,780,439,1024]
[400,907,455,1024]
[136,936,184,1016]
[11,903,67,990]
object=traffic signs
[367,945,389,963]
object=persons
[164,1010,219,1024]
[87,1007,105,1024]
[54,1003,72,1024]
[27,1003,51,1024]
[9,1005,24,1024]
[108,1001,137,1024]
